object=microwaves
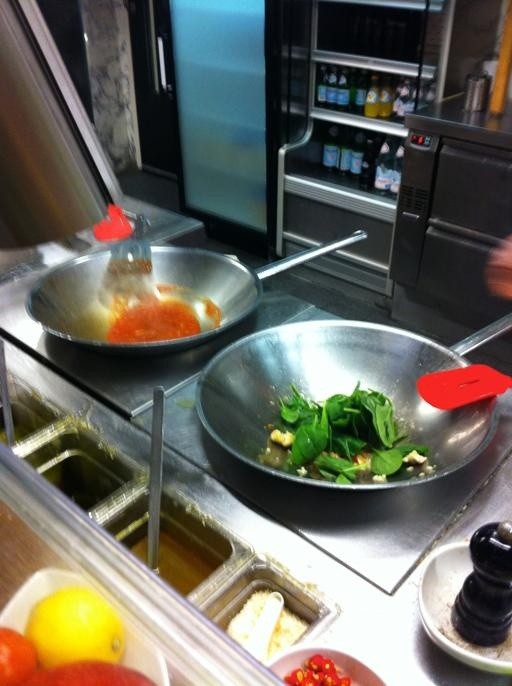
[309,0,455,78]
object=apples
[21,661,158,686]
[1,629,37,686]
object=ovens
[389,111,512,371]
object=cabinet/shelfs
[274,1,503,296]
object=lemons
[25,584,128,671]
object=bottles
[312,63,435,200]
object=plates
[419,537,512,680]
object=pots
[193,318,497,494]
[25,227,371,350]
